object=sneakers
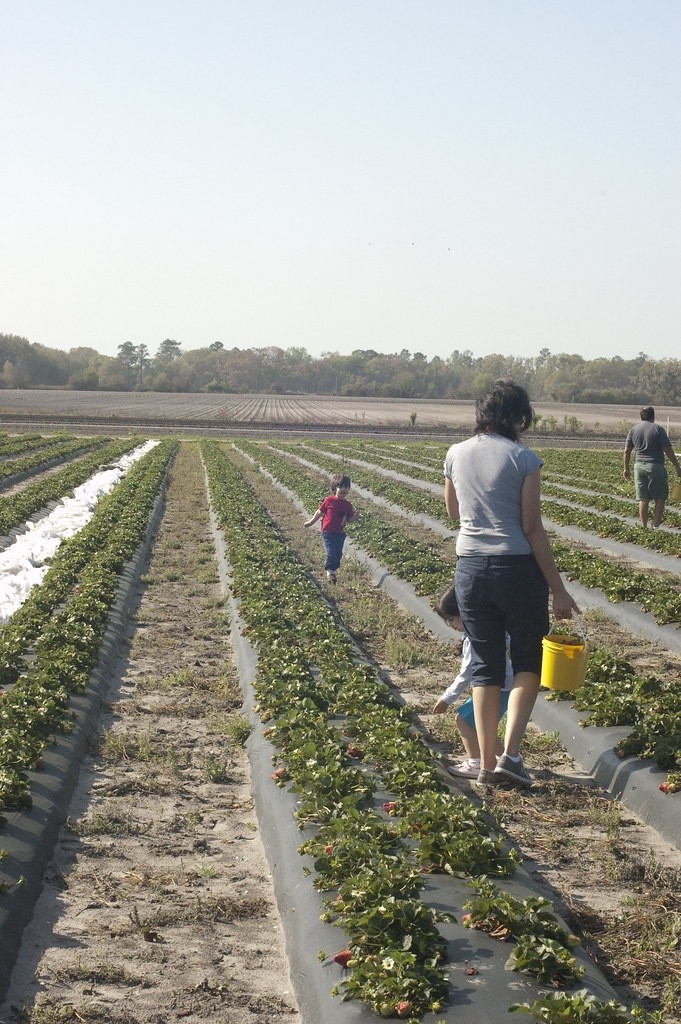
[447,759,479,779]
[476,769,512,784]
[493,754,532,786]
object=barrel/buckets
[671,476,681,500]
[540,610,588,690]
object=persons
[622,405,681,532]
[432,580,517,779]
[444,380,582,788]
[303,474,360,586]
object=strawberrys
[254,694,582,1017]
[658,782,678,793]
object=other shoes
[327,573,337,582]
